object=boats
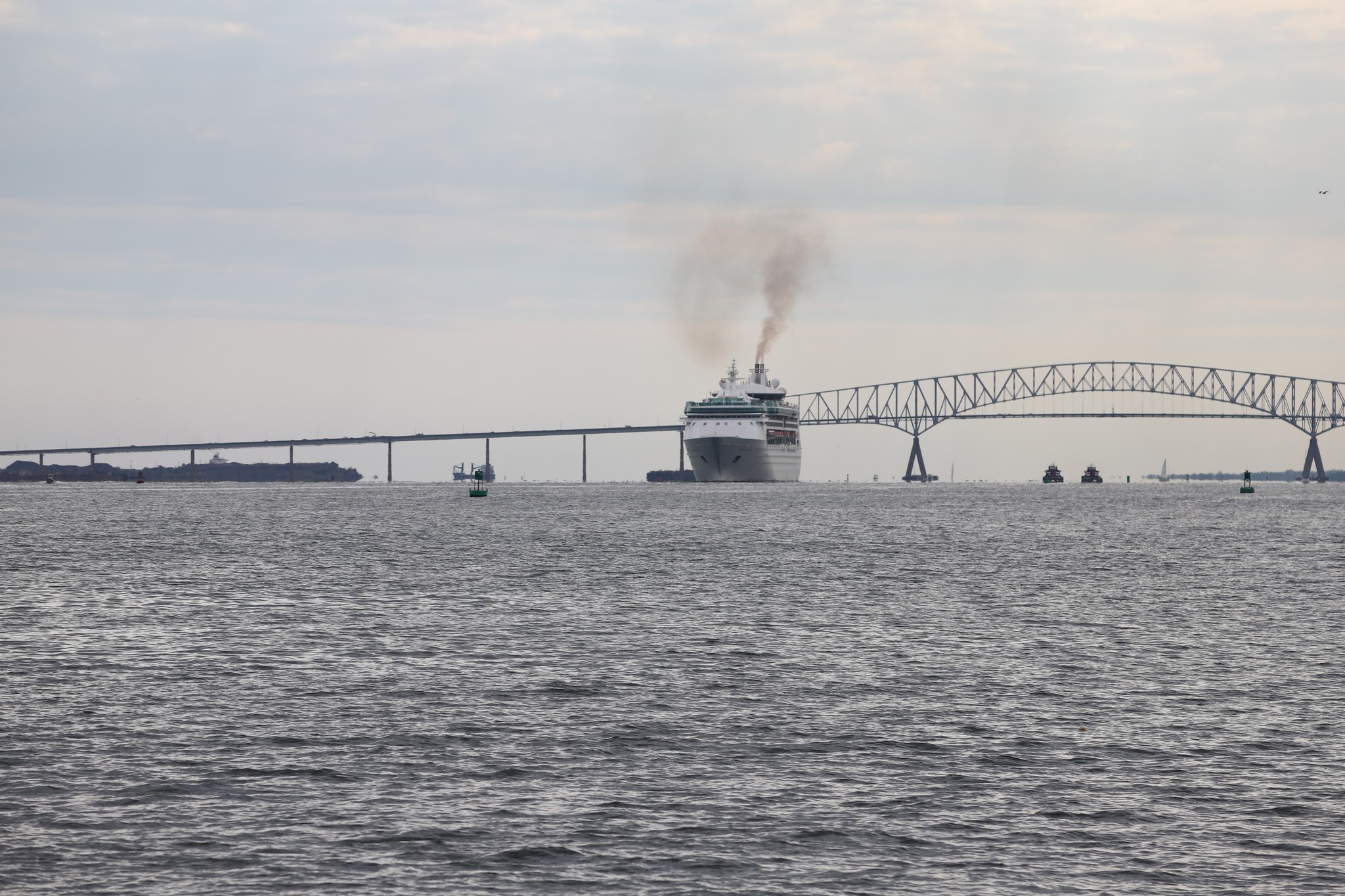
[1296,475,1330,482]
[1042,462,1064,483]
[646,469,697,483]
[45,474,56,483]
[1081,464,1103,484]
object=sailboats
[1159,458,1170,482]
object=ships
[678,359,801,482]
